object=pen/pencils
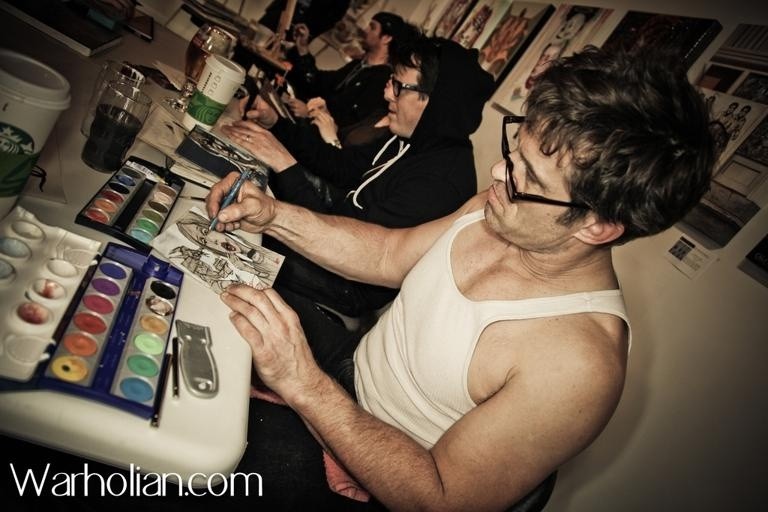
[242,90,257,123]
[152,353,171,426]
[207,168,252,234]
[173,337,179,396]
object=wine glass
[162,24,237,114]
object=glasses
[501,115,594,210]
[391,72,429,97]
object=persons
[177,0,750,512]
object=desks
[1,1,266,489]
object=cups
[181,53,247,135]
[0,50,72,222]
[81,81,152,174]
[80,61,145,137]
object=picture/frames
[432,0,479,41]
[479,2,556,101]
[601,9,723,74]
